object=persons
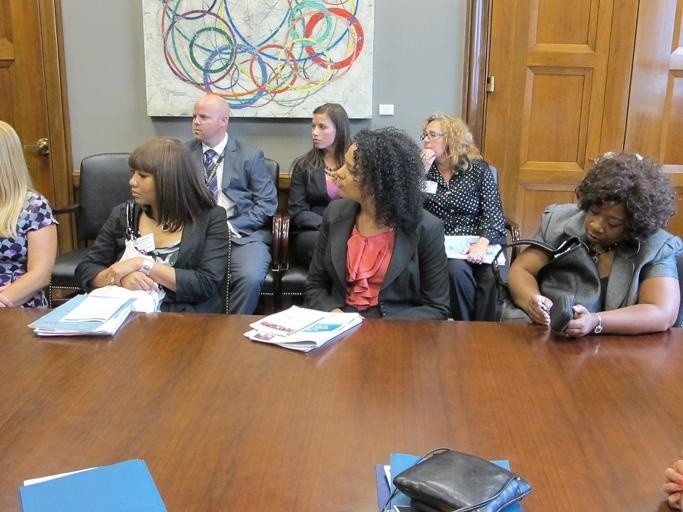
[75,136,228,315]
[420,113,507,322]
[289,103,354,268]
[305,126,450,320]
[183,94,278,315]
[664,460,683,512]
[0,120,59,309]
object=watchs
[595,313,603,334]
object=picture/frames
[139,2,373,117]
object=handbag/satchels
[383,448,532,512]
[490,234,601,340]
[112,199,156,265]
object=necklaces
[321,155,346,177]
[146,209,184,232]
[588,240,620,264]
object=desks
[0,305,683,512]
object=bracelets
[140,258,154,275]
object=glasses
[419,131,444,139]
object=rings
[111,278,115,282]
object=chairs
[258,157,281,310]
[282,188,321,308]
[479,163,526,322]
[24,151,139,302]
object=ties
[204,151,218,205]
